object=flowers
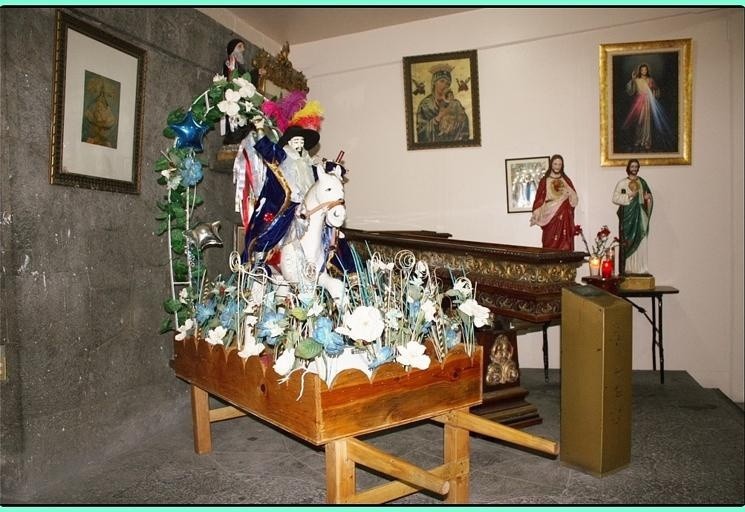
[574,225,618,256]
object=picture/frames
[403,50,480,150]
[232,223,246,253]
[505,156,551,213]
[599,37,693,166]
[49,10,146,194]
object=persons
[529,155,578,252]
[411,63,471,142]
[512,167,546,207]
[223,39,257,145]
[612,160,653,277]
[251,115,346,278]
[620,64,661,150]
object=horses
[251,161,351,324]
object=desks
[619,286,679,383]
[170,337,484,505]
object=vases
[589,253,601,276]
